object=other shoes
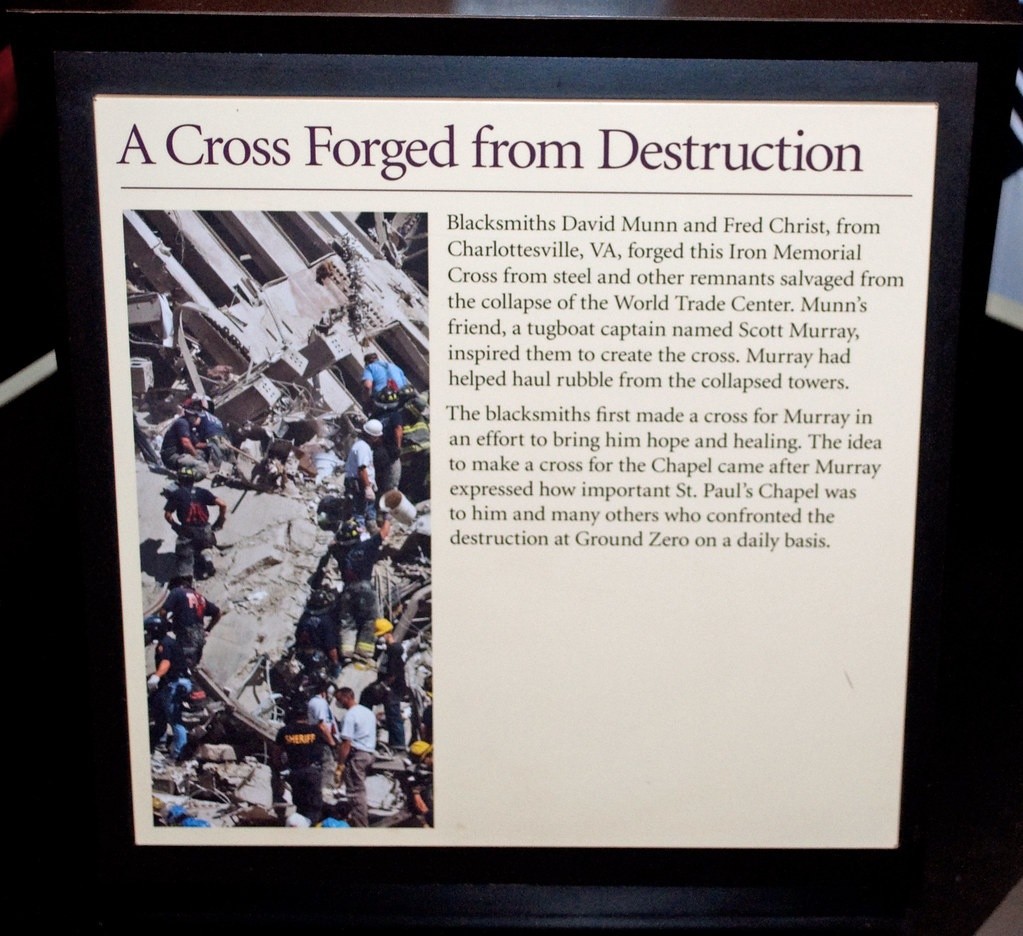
[342,653,377,666]
[206,560,216,577]
[389,745,407,752]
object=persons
[136,307,439,831]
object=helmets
[375,387,399,410]
[144,616,161,629]
[177,466,195,480]
[398,385,417,397]
[167,577,191,587]
[363,419,384,438]
[305,589,336,614]
[184,402,206,417]
[410,740,433,760]
[336,520,363,547]
[373,617,394,638]
